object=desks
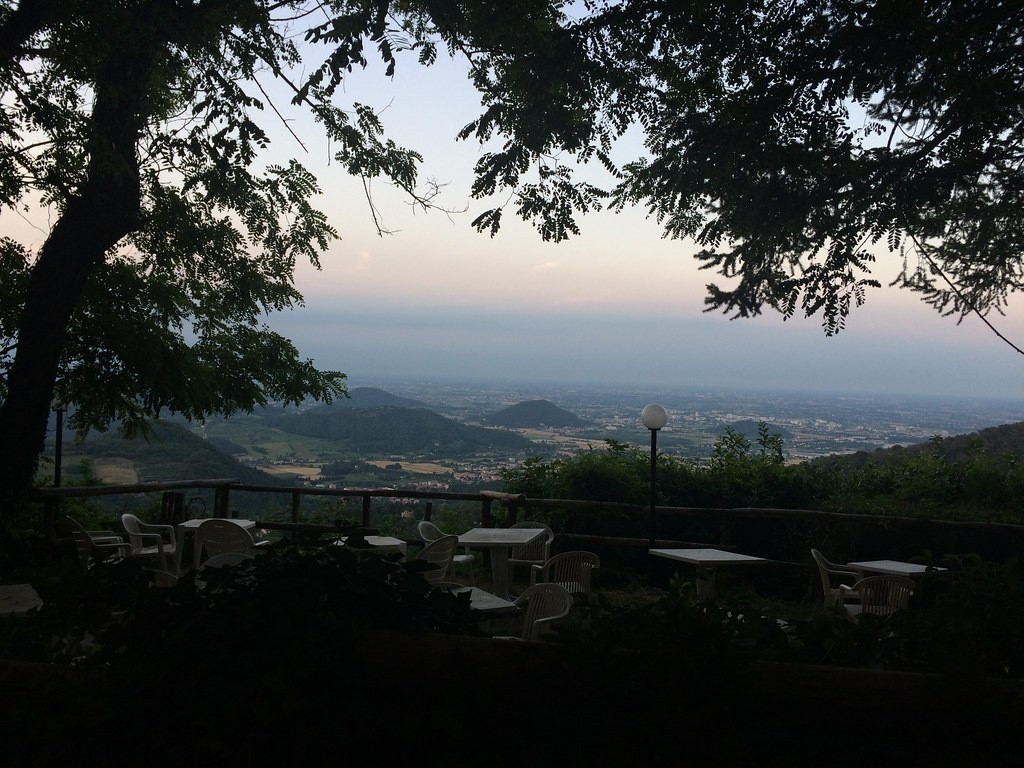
[648,548,767,610]
[457,527,546,615]
[439,586,516,635]
[329,536,406,563]
[847,560,945,578]
[174,519,255,578]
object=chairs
[65,513,947,645]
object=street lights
[52,388,70,488]
[642,406,668,549]
